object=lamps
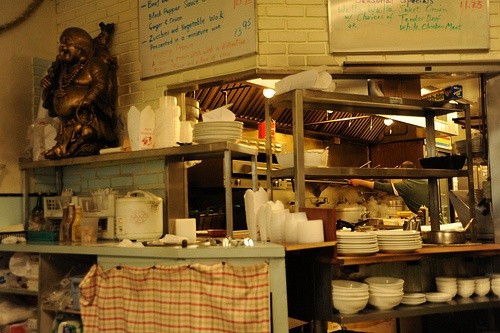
[326,111,333,121]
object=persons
[39,27,117,159]
[350,160,445,225]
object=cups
[78,217,99,244]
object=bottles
[58,203,84,243]
[32,195,44,224]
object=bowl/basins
[331,276,405,315]
[419,155,466,170]
[452,137,481,154]
[427,231,464,244]
[434,273,500,299]
[275,149,328,167]
[208,231,227,237]
[266,208,307,243]
[154,96,181,148]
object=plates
[401,293,451,305]
[335,230,422,256]
[192,121,243,143]
[297,220,324,243]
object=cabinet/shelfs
[0,88,500,333]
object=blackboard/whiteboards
[327,0,491,54]
[135,0,259,81]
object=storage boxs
[26,230,59,242]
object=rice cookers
[336,205,367,222]
[113,190,163,242]
